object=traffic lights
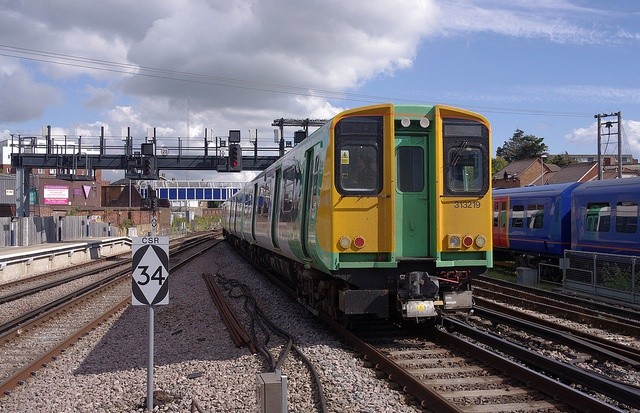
[228,143,243,172]
[142,156,155,175]
[152,198,157,211]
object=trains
[492,176,640,267]
[220,103,494,329]
